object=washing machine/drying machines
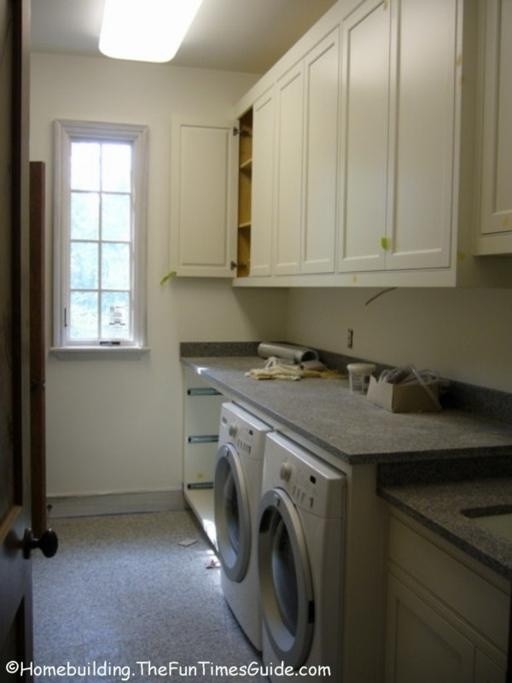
[215,403,271,652]
[257,433,344,683]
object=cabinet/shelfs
[375,474,512,681]
[214,88,272,278]
[338,0,458,274]
[476,4,512,253]
[273,27,340,276]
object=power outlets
[347,329,353,349]
